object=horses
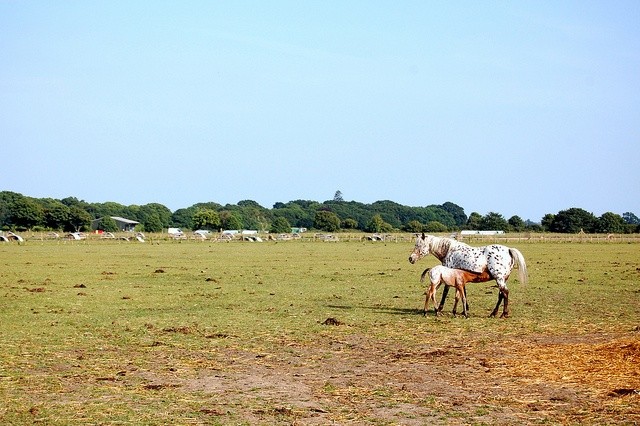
[419,264,495,318]
[409,232,527,319]
